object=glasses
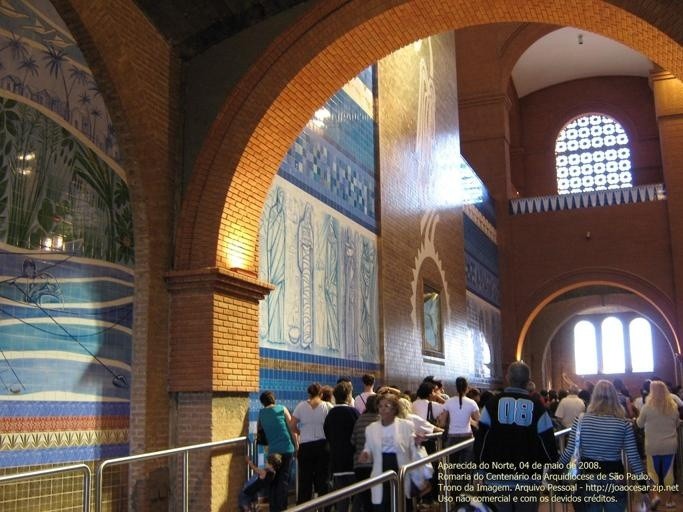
[377,403,391,409]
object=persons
[324,381,357,485]
[398,398,445,480]
[375,385,417,403]
[545,380,649,512]
[474,360,570,511]
[578,390,590,404]
[413,376,450,423]
[351,395,381,511]
[238,453,282,512]
[354,374,376,414]
[558,390,566,400]
[292,385,329,501]
[554,384,585,455]
[540,391,547,398]
[257,391,299,511]
[623,389,629,396]
[634,376,682,407]
[544,390,559,408]
[337,375,355,407]
[359,394,414,512]
[466,388,480,403]
[582,380,593,394]
[320,385,334,403]
[612,377,632,418]
[448,377,480,463]
[636,383,679,508]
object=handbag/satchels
[256,418,269,446]
[423,401,440,442]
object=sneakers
[666,500,678,508]
[652,495,661,508]
